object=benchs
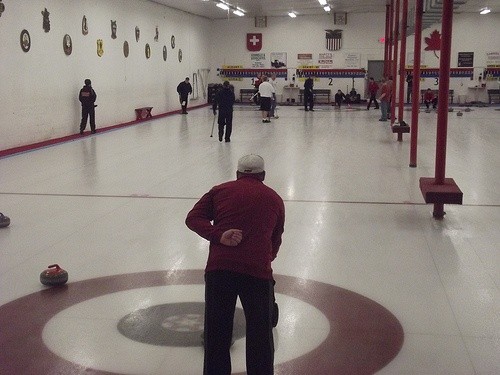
[134,106,154,121]
[420,90,454,105]
[239,89,274,103]
[487,89,500,105]
[298,89,331,104]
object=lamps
[319,0,331,11]
[215,0,246,16]
[287,11,297,18]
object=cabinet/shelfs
[208,84,215,104]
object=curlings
[465,108,470,112]
[0,212,10,227]
[425,109,431,112]
[40,263,68,285]
[449,108,453,112]
[457,111,463,116]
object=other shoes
[379,119,387,121]
[367,107,369,110]
[263,120,271,123]
[225,138,230,142]
[374,108,379,109]
[219,138,222,141]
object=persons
[304,74,315,112]
[251,73,263,106]
[334,90,350,108]
[407,71,413,105]
[366,77,379,110]
[350,88,358,103]
[424,88,439,109]
[258,75,274,123]
[269,74,277,118]
[78,79,97,134]
[213,81,235,142]
[185,153,285,375]
[379,75,397,122]
[177,77,192,114]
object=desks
[468,87,484,104]
[284,87,300,105]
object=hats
[237,154,264,173]
